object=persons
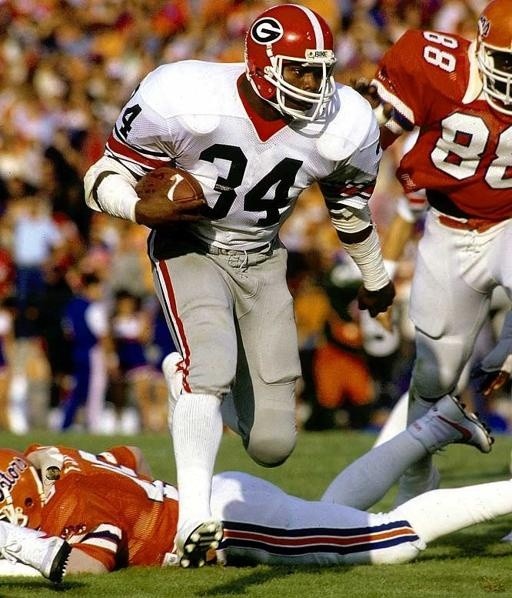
[0,1,512,434]
[0,394,512,576]
[84,4,396,567]
[351,0,512,512]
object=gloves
[346,77,392,128]
[135,176,206,224]
[359,281,396,317]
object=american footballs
[136,168,204,215]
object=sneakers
[175,520,226,570]
[396,455,441,508]
[406,394,495,453]
[161,349,186,439]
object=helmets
[244,4,335,100]
[0,448,43,528]
[475,0,512,82]
